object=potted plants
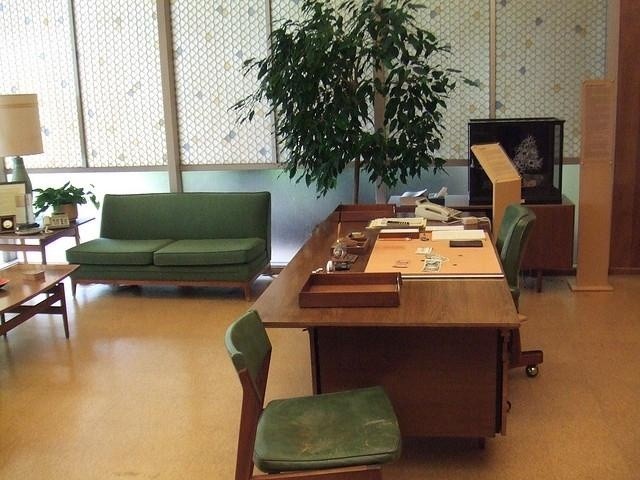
[31,183,101,224]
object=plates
[15,228,43,236]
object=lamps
[0,92,44,228]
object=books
[400,189,428,205]
[369,218,427,230]
[432,229,486,241]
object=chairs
[222,310,402,477]
[484,205,544,378]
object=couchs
[64,193,273,303]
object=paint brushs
[337,213,342,241]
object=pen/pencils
[388,221,410,224]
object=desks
[240,201,523,450]
[387,195,575,294]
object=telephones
[44,211,70,229]
[415,197,463,221]
